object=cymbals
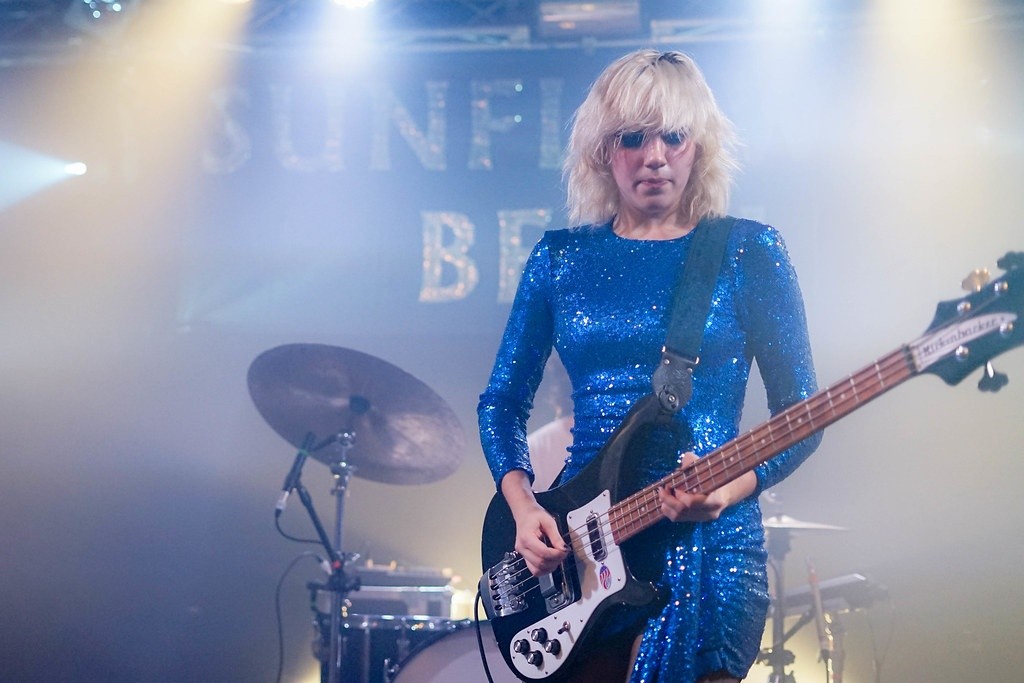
[246,344,467,487]
[761,514,849,533]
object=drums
[312,609,484,683]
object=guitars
[480,251,1024,683]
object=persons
[476,45,826,682]
